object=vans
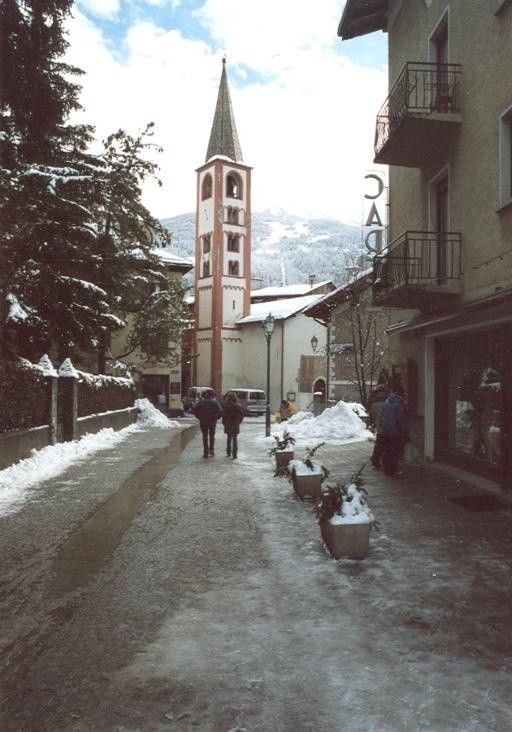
[221,388,267,416]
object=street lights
[261,313,276,437]
[310,335,330,406]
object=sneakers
[228,453,237,458]
[203,453,215,458]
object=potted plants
[263,427,382,558]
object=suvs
[183,386,217,412]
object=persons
[194,390,244,458]
[279,399,296,420]
[369,386,409,479]
